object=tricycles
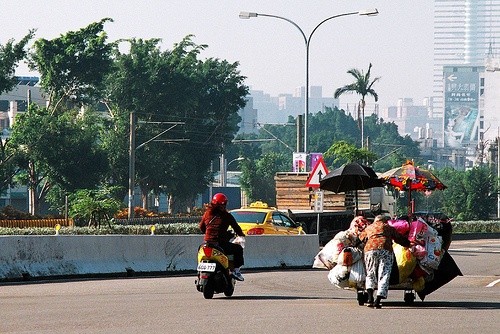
[414,211,452,252]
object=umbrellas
[320,163,383,216]
[378,160,448,214]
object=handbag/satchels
[387,219,409,235]
[407,220,428,246]
[419,235,443,270]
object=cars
[226,201,307,235]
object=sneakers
[231,270,244,281]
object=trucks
[278,173,398,246]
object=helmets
[211,192,228,206]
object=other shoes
[366,300,373,307]
[372,302,382,309]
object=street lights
[239,7,379,153]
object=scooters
[194,229,243,299]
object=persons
[359,215,414,309]
[198,193,246,282]
